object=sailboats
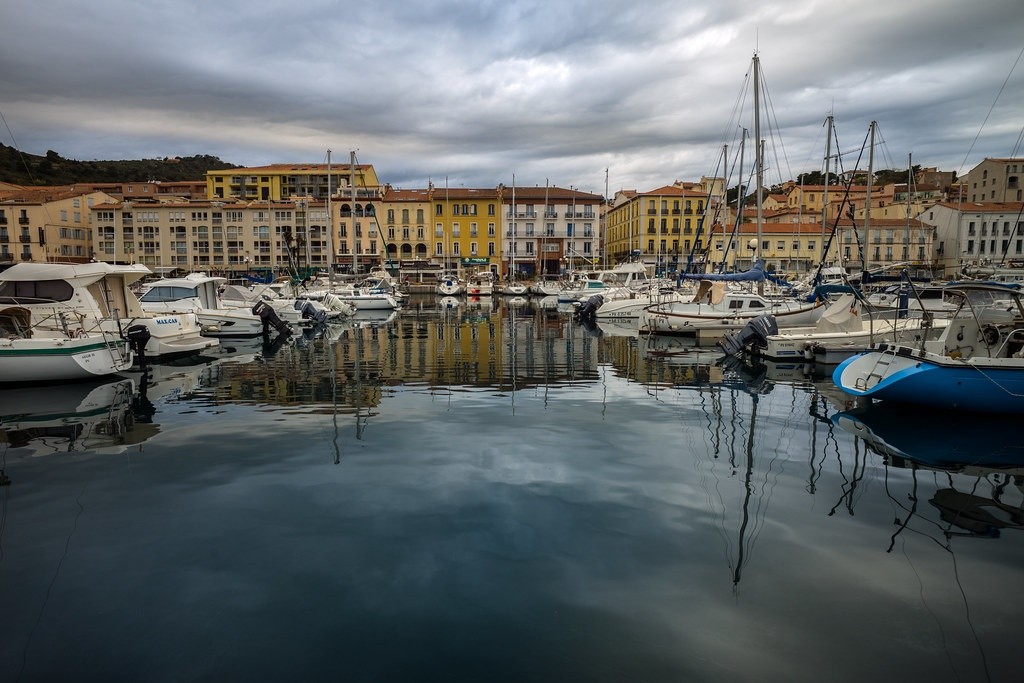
[437,50,1023,417]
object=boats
[1,261,405,382]
